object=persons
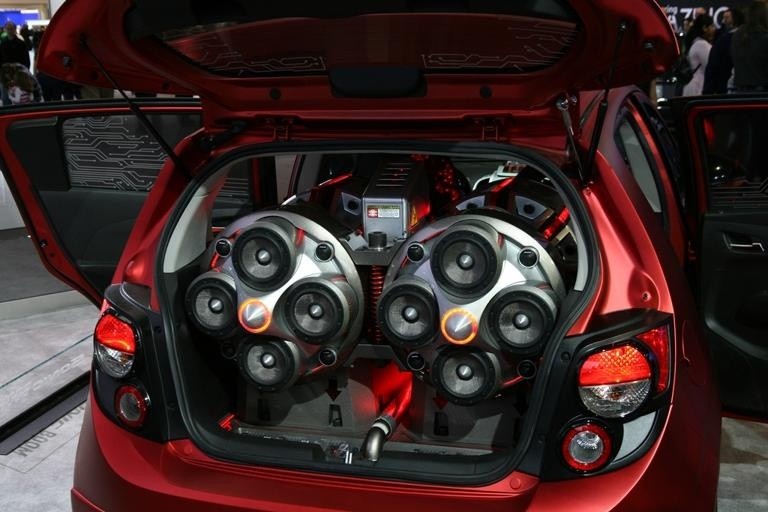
[641,4,768,190]
[1,21,115,104]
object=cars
[0,1,767,512]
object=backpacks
[677,39,702,85]
